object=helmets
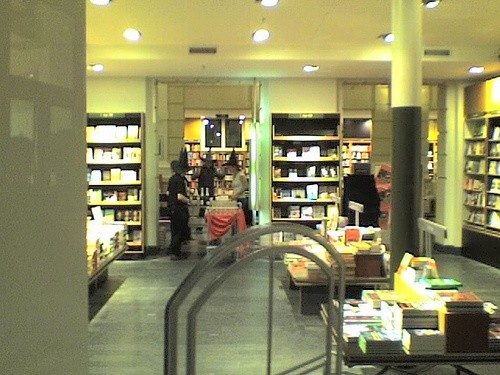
[171,147,190,172]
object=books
[468,123,484,137]
[465,177,482,205]
[488,178,500,227]
[343,144,371,175]
[86,168,138,203]
[274,146,337,177]
[466,141,485,173]
[468,208,483,223]
[184,168,249,196]
[488,127,500,174]
[86,206,142,274]
[184,144,250,166]
[86,125,141,163]
[273,219,390,278]
[274,184,340,218]
[333,253,500,353]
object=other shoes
[172,251,191,261]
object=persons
[222,151,250,226]
[166,148,193,261]
[191,150,225,195]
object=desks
[281,253,390,315]
[320,300,500,375]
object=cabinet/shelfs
[343,116,372,176]
[427,140,438,179]
[184,140,250,205]
[86,112,145,260]
[462,115,500,270]
[271,112,343,260]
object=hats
[200,147,218,162]
[222,151,240,168]
